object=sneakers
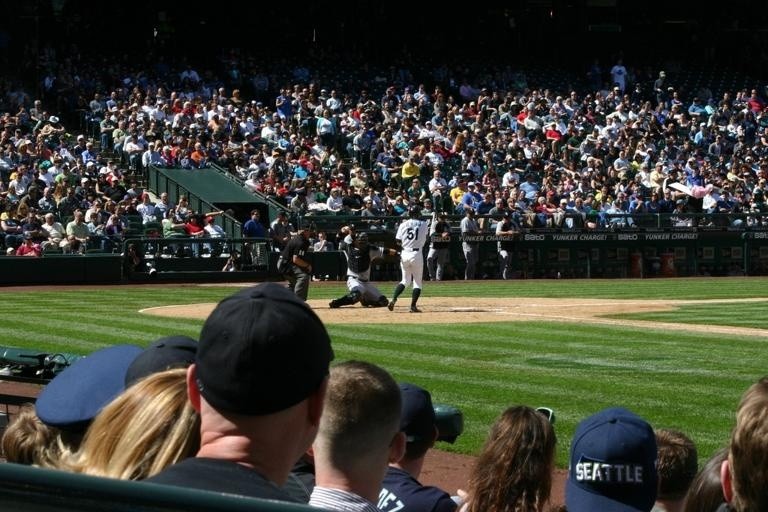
[409,307,421,313]
[388,302,394,311]
[329,300,338,308]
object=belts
[413,248,419,251]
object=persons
[387,206,429,313]
[139,282,333,505]
[285,360,767,510]
[276,221,312,301]
[329,225,399,308]
[0,50,766,279]
[1,332,201,512]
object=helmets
[352,231,368,249]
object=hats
[36,344,144,430]
[195,283,332,415]
[565,407,659,512]
[125,335,198,390]
[4,102,217,240]
[398,384,436,442]
